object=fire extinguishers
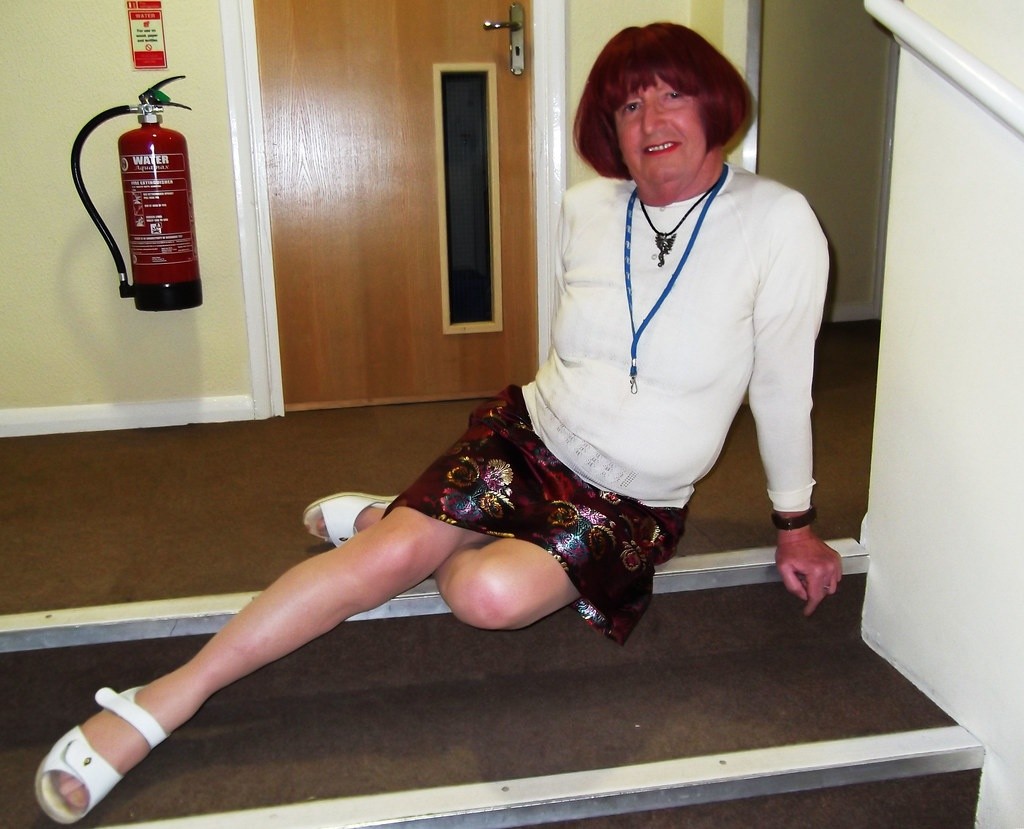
[117,74,204,312]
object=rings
[824,585,831,592]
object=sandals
[303,492,401,548]
[34,684,172,825]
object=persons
[35,21,843,823]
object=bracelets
[771,504,817,530]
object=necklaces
[639,165,723,266]
